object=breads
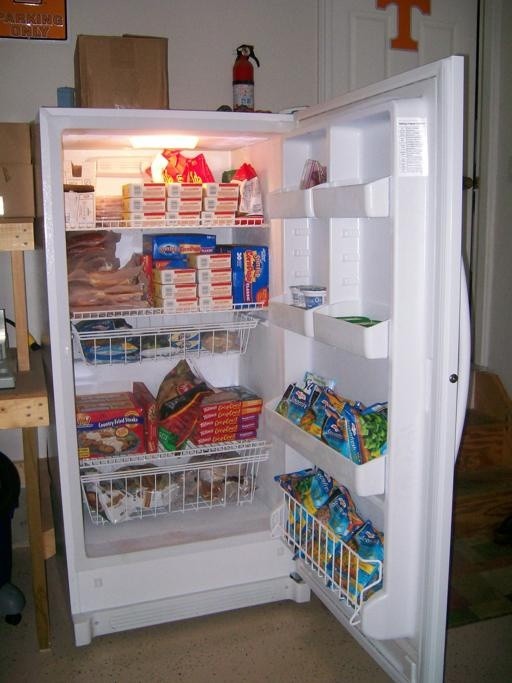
[68,231,149,314]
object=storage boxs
[74,34,170,109]
[0,163,34,219]
[0,122,32,164]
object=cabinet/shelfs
[1,217,56,651]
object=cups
[56,88,73,107]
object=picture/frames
[0,0,67,41]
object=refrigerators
[40,54,476,682]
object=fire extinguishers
[232,45,260,112]
